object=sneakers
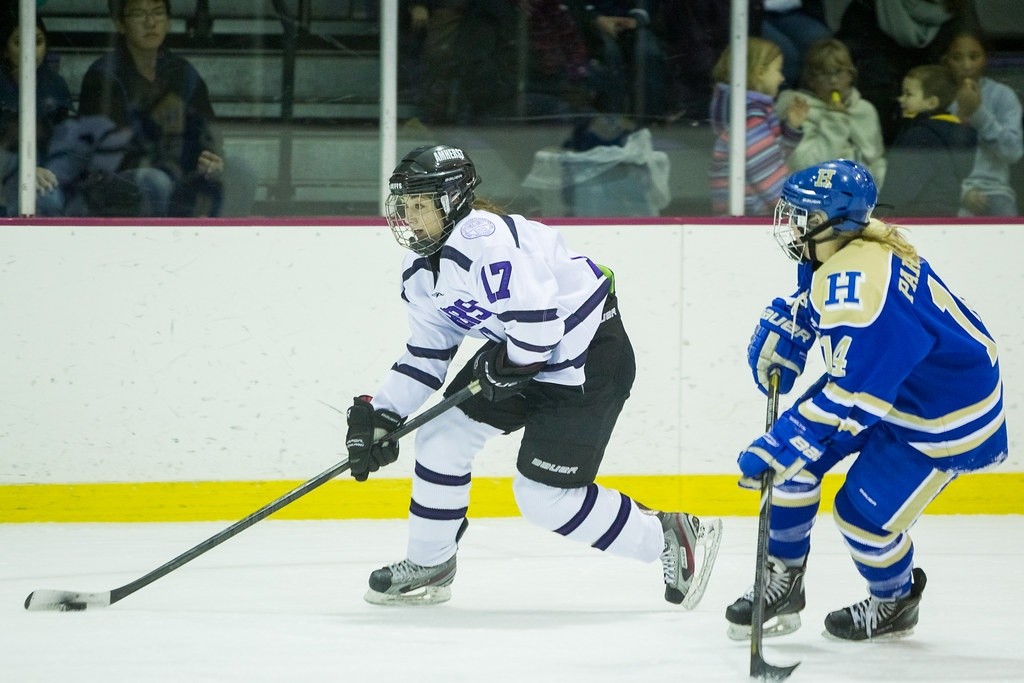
[659,512,723,610]
[725,556,807,641]
[363,548,458,607]
[823,568,928,643]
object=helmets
[774,158,895,265]
[385,144,483,257]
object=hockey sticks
[22,378,483,611]
[748,366,802,682]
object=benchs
[2,0,688,214]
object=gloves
[747,298,817,397]
[474,340,548,403]
[737,411,826,491]
[345,394,408,483]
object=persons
[346,144,723,612]
[709,37,808,216]
[725,159,1008,641]
[871,65,977,218]
[78,0,224,218]
[775,38,887,200]
[409,0,963,129]
[0,8,77,220]
[942,19,1024,217]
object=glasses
[120,8,170,24]
[807,64,856,81]
